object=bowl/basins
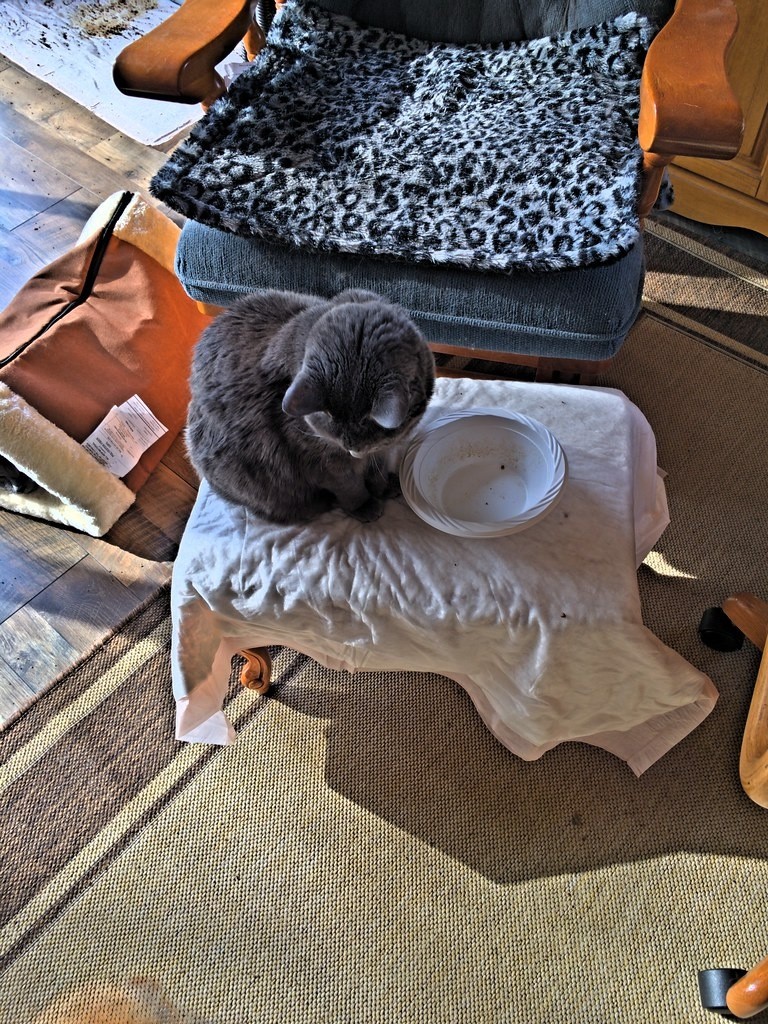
[398,406,569,537]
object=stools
[169,376,721,777]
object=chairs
[111,0,745,386]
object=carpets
[0,214,768,1024]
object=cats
[182,287,435,525]
